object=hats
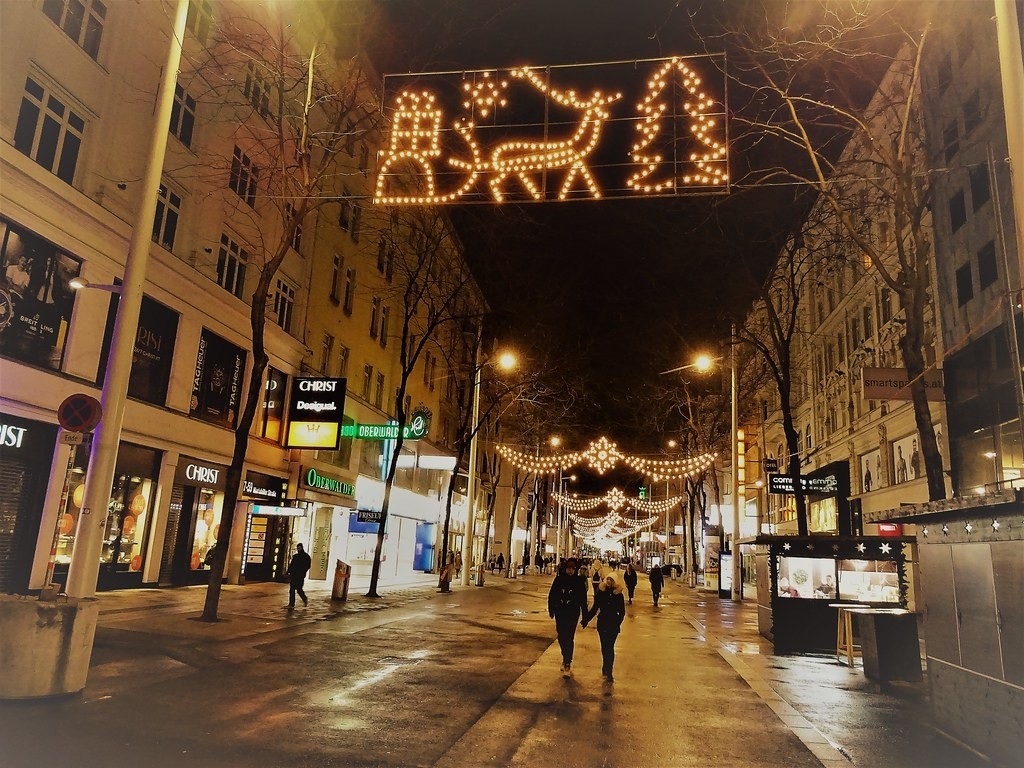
[606,572,618,583]
[566,560,577,570]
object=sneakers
[563,667,570,678]
[560,662,565,671]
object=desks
[844,606,922,681]
[829,602,871,667]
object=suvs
[660,564,682,577]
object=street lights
[459,323,516,586]
[527,417,561,575]
[60,277,141,698]
[693,322,745,602]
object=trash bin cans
[508,561,517,579]
[688,569,696,587]
[331,559,352,602]
[475,562,485,586]
[548,562,555,574]
[671,567,678,579]
[842,606,924,697]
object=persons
[580,573,625,682]
[624,564,637,603]
[284,543,311,608]
[455,554,462,578]
[864,430,943,493]
[535,551,553,574]
[814,575,836,598]
[779,577,800,597]
[649,565,664,606]
[548,558,589,679]
[576,558,592,591]
[599,556,633,571]
[490,553,504,574]
[589,560,605,595]
[0,256,59,346]
